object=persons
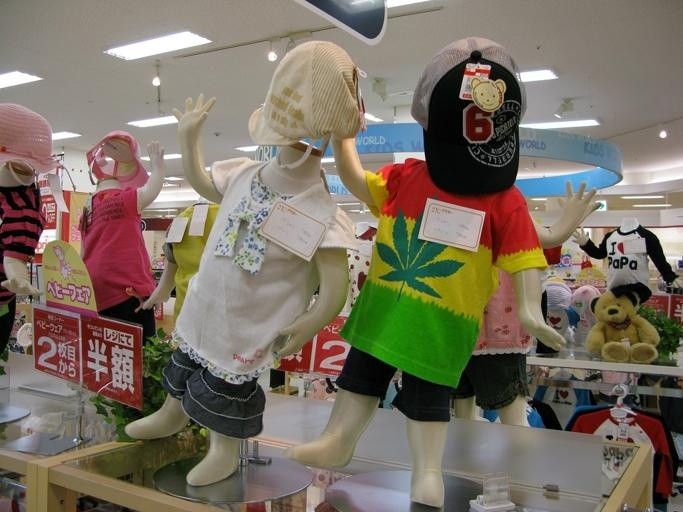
[570,216,683,291]
[0,101,53,370]
[79,141,166,346]
[283,37,566,509]
[125,40,361,488]
[447,180,603,429]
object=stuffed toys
[539,276,663,364]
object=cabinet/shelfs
[1,352,657,509]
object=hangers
[608,385,636,422]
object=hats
[248,41,368,146]
[86,129,149,188]
[410,37,528,195]
[0,103,62,174]
